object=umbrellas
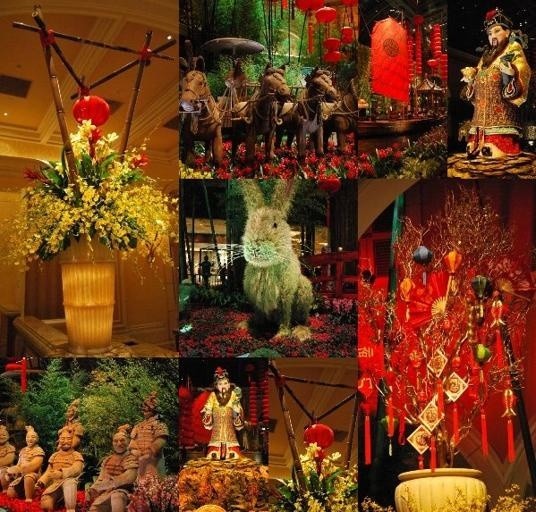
[199,37,266,60]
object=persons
[453,7,533,160]
[34,426,85,512]
[5,424,46,504]
[127,396,170,488]
[54,397,86,454]
[0,424,19,494]
[226,59,249,102]
[197,366,245,462]
[199,255,214,282]
[85,423,140,511]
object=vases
[59,230,117,355]
[394,467,488,512]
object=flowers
[1,118,179,291]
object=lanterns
[248,374,270,429]
[321,36,343,53]
[315,177,342,227]
[303,424,338,476]
[339,27,354,37]
[412,15,449,86]
[70,94,109,156]
[295,1,325,55]
[323,51,342,65]
[342,36,354,45]
[313,7,338,40]
[177,384,195,451]
